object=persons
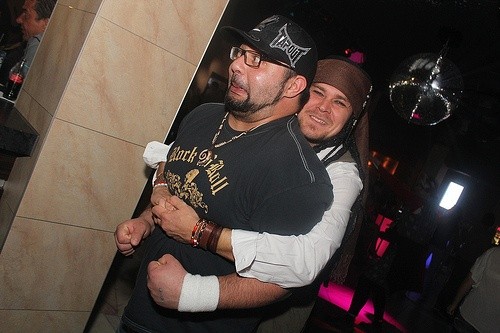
[16,0,58,76]
[143,56,372,333]
[330,161,435,333]
[114,15,334,333]
[447,247,500,333]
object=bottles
[2,56,26,101]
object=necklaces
[208,112,269,150]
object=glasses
[228,45,297,72]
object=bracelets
[156,171,163,177]
[190,219,223,254]
[178,273,219,312]
[153,182,168,191]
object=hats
[221,14,318,87]
